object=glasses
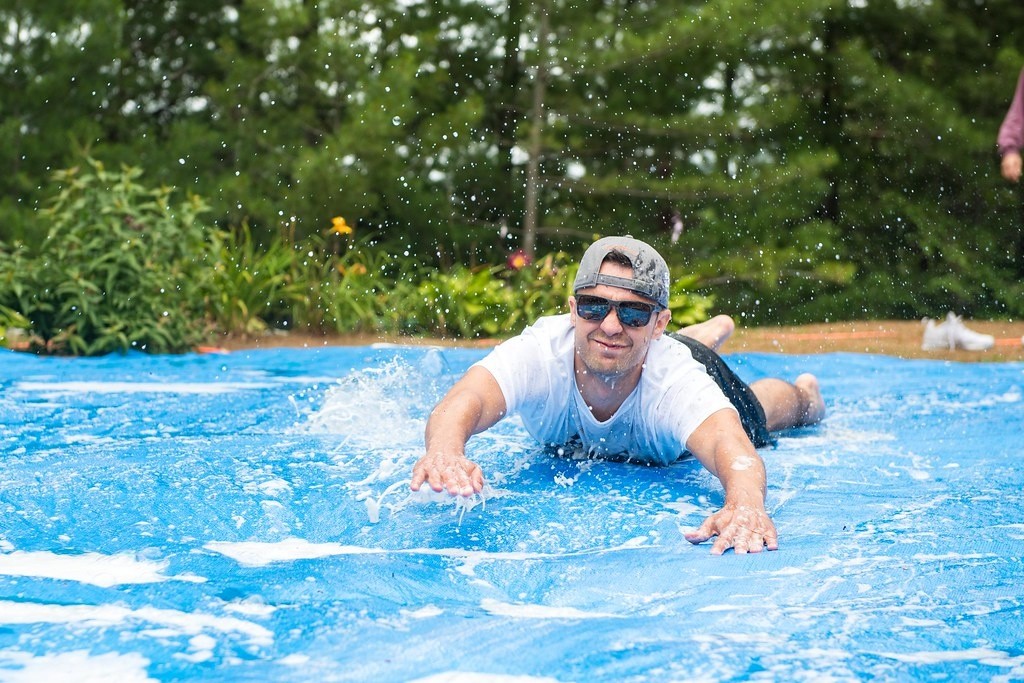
[573,293,662,327]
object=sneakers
[920,312,995,353]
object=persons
[410,232,825,555]
[996,62,1024,182]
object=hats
[573,234,670,309]
